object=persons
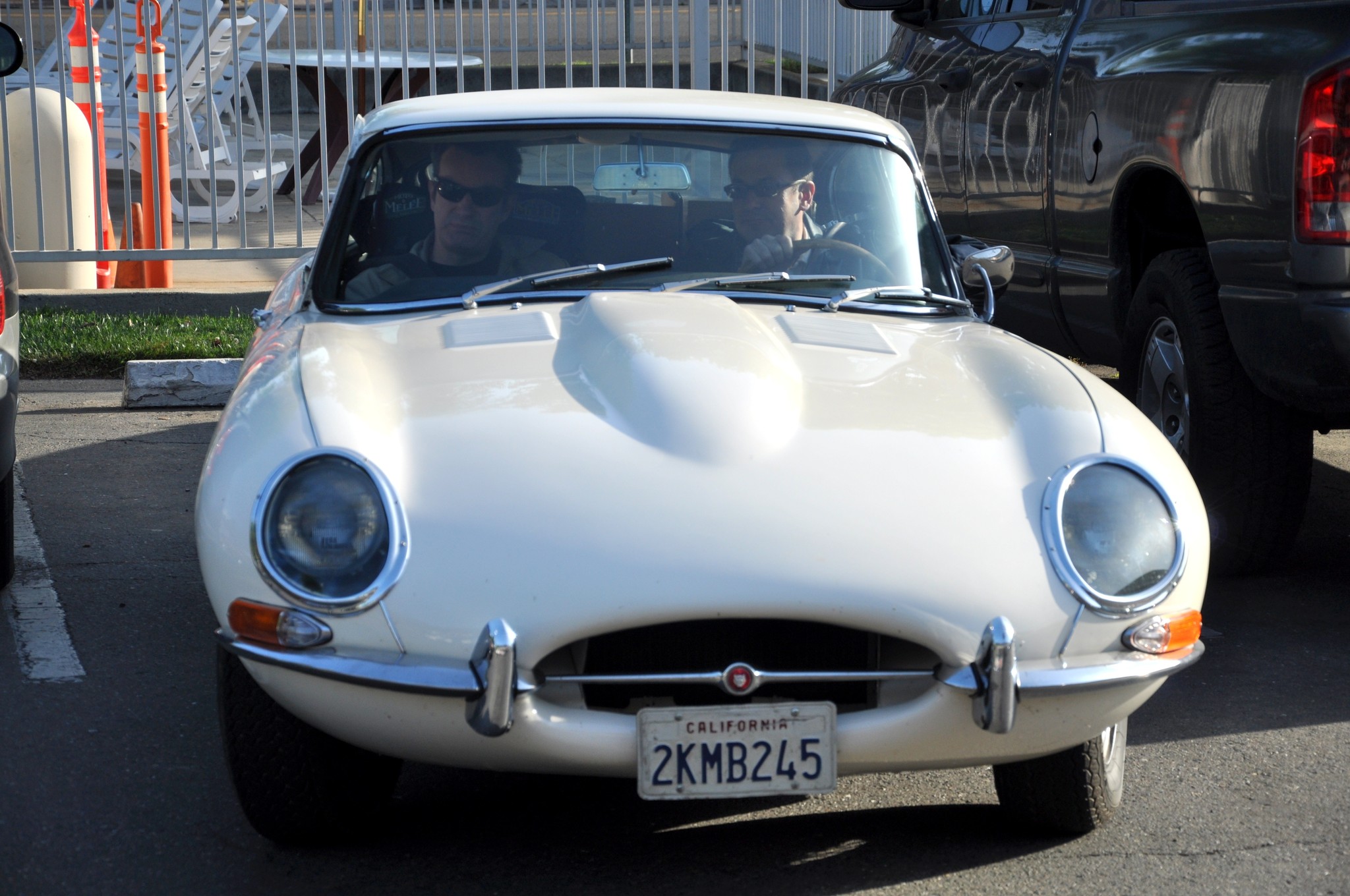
[343,141,572,305]
[728,134,891,279]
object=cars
[820,1,1350,497]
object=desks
[238,43,483,207]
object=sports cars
[194,86,1214,847]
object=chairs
[0,0,311,229]
[502,185,737,264]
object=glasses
[723,177,806,199]
[433,177,506,207]
